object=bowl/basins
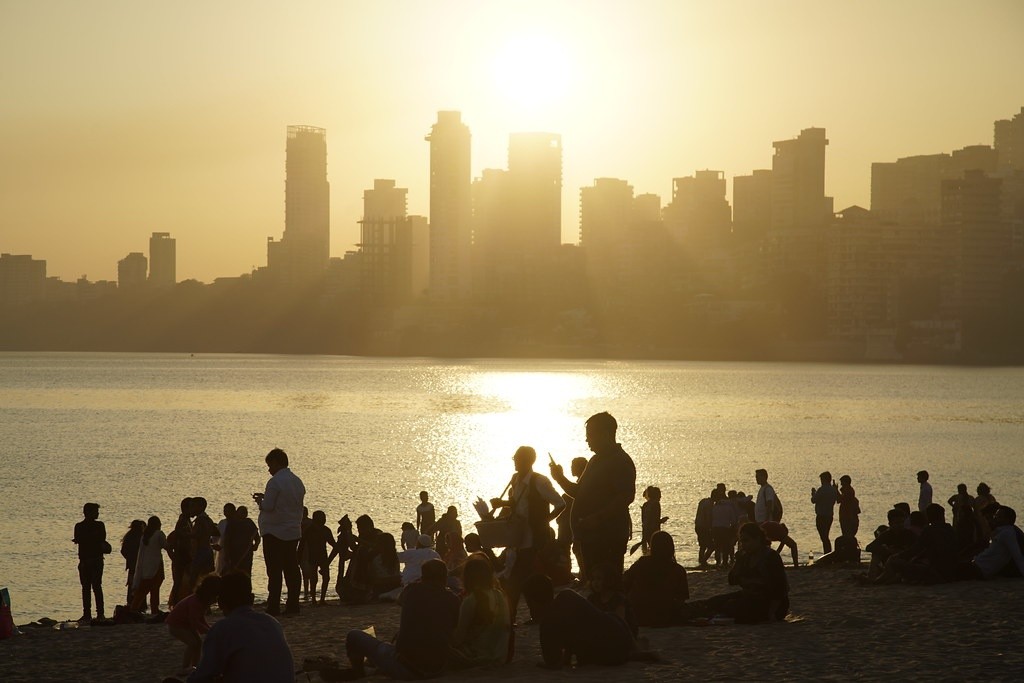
[474,520,523,548]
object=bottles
[52,622,80,630]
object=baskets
[475,516,523,549]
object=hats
[417,534,432,546]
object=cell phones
[549,453,556,466]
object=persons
[166,573,223,669]
[297,492,516,677]
[557,457,588,578]
[253,448,305,612]
[586,468,1024,643]
[73,497,261,621]
[491,447,567,628]
[161,569,295,683]
[550,413,637,580]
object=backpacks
[336,571,374,606]
